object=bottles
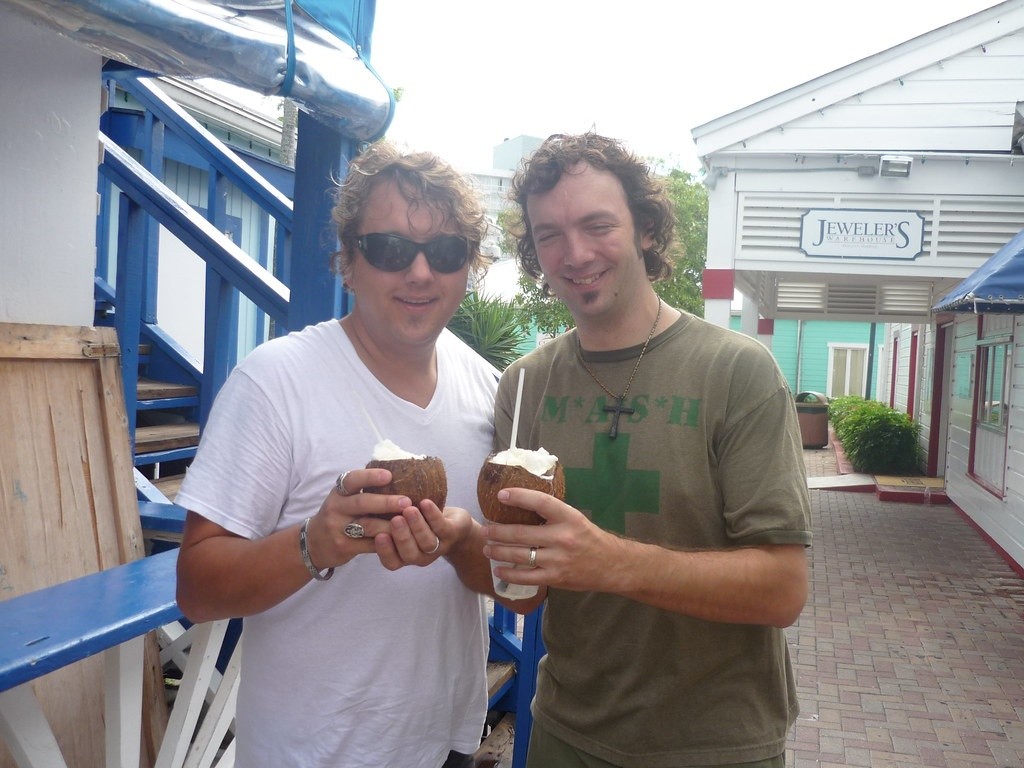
[924,486,930,504]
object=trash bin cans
[794,391,830,448]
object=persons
[175,147,504,768]
[376,136,811,768]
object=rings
[343,520,367,543]
[426,536,440,554]
[336,466,350,495]
[527,546,538,568]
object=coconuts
[476,445,566,525]
[362,440,447,523]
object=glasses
[352,232,473,273]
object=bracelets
[300,515,334,586]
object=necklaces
[570,294,663,443]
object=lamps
[879,156,912,178]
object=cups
[488,516,539,600]
